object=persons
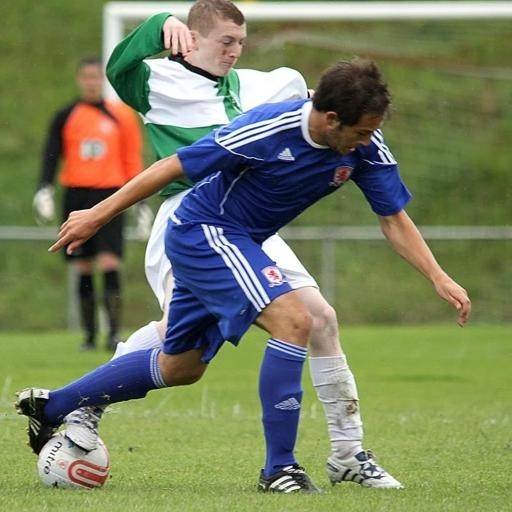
[65,0,405,491]
[15,58,471,493]
[32,58,145,350]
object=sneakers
[64,402,104,453]
[325,447,405,490]
[13,386,63,456]
[77,336,123,350]
[257,463,323,495]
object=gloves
[122,202,155,245]
[30,185,57,228]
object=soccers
[37,428,111,490]
